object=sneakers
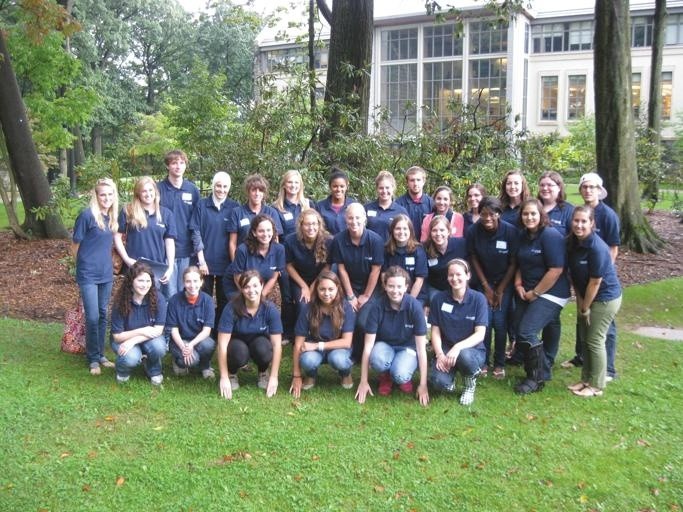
[561,356,613,397]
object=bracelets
[578,308,591,317]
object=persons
[155,150,569,404]
[567,205,622,399]
[73,178,137,377]
[111,267,167,386]
[560,172,620,384]
[112,176,176,294]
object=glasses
[583,185,598,189]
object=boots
[446,370,481,405]
[513,339,544,393]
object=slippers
[480,366,505,380]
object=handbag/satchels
[61,309,87,354]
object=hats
[579,173,607,200]
